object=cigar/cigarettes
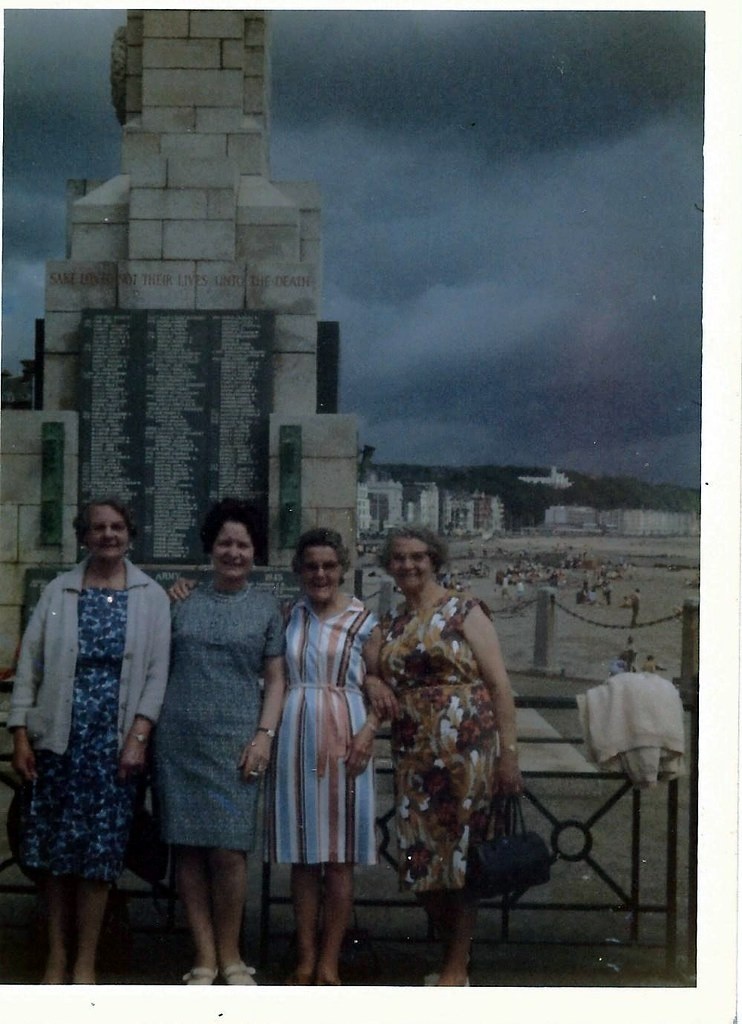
[250,771,258,776]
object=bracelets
[367,722,378,733]
[500,743,518,752]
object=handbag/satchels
[465,795,550,899]
[6,780,45,884]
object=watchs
[130,732,146,743]
[259,727,275,738]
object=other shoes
[217,964,257,985]
[283,958,319,986]
[183,963,219,985]
[424,950,470,987]
[315,966,341,986]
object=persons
[631,589,641,616]
[643,655,656,673]
[436,539,625,606]
[147,497,285,986]
[5,494,172,985]
[167,528,390,986]
[610,636,636,678]
[365,522,521,986]
